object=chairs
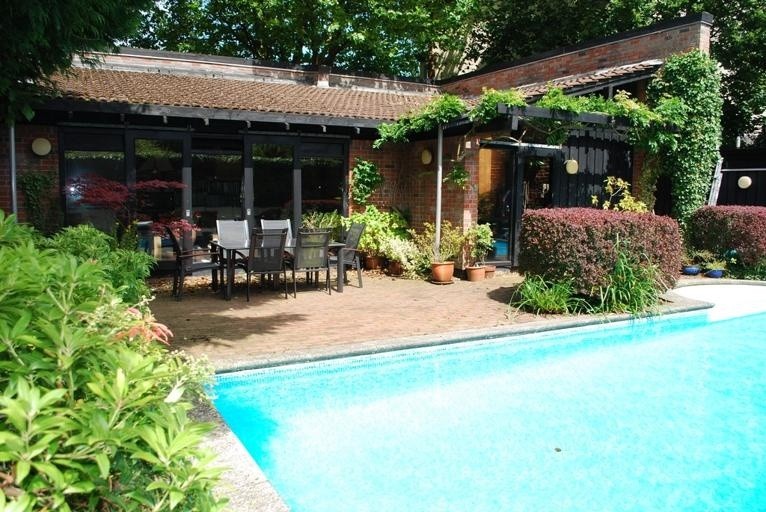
[165,219,366,302]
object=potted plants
[361,203,410,277]
[682,259,727,278]
[411,219,496,285]
